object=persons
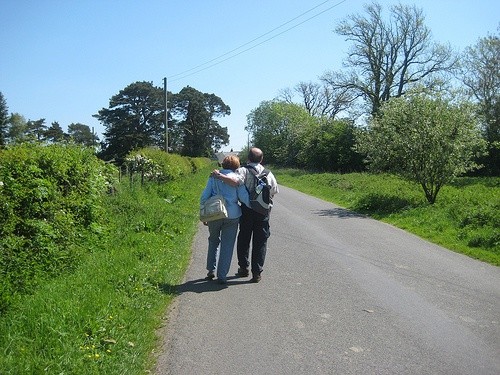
[212,148,279,283]
[200,155,269,284]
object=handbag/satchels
[199,194,228,222]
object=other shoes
[251,272,262,282]
[235,269,249,277]
[218,278,226,284]
[207,272,215,278]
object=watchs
[217,173,221,177]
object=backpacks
[241,163,272,218]
